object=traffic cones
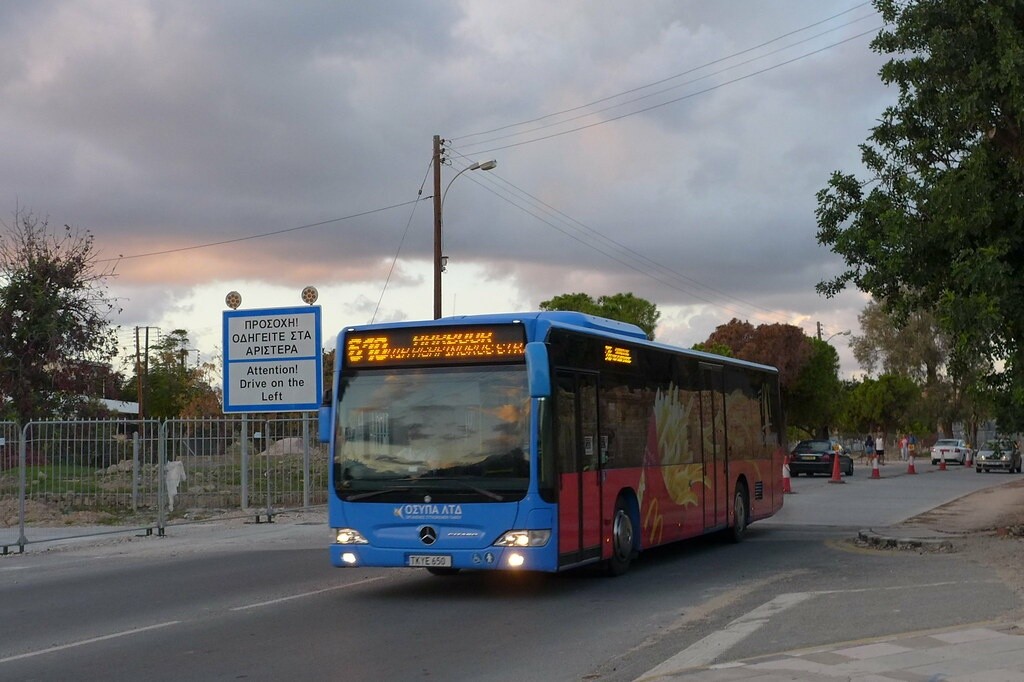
[867,451,884,479]
[937,450,949,471]
[903,450,918,474]
[964,450,973,468]
[827,451,845,484]
[783,456,797,494]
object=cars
[976,438,1022,474]
[788,439,853,478]
[931,438,974,465]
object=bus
[317,310,785,580]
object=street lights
[433,159,499,320]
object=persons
[899,432,914,461]
[865,435,885,467]
